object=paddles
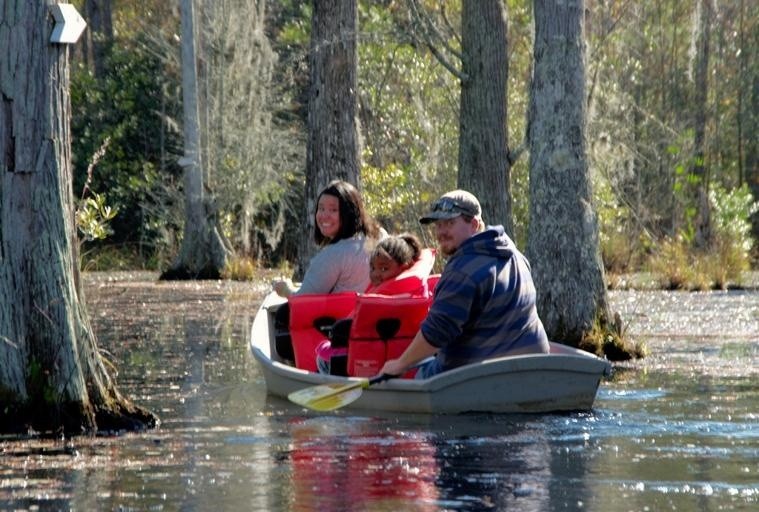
[287,355,436,412]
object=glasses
[430,197,476,214]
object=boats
[250,281,609,415]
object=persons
[316,233,428,377]
[378,189,550,380]
[270,180,389,363]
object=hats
[419,190,483,225]
